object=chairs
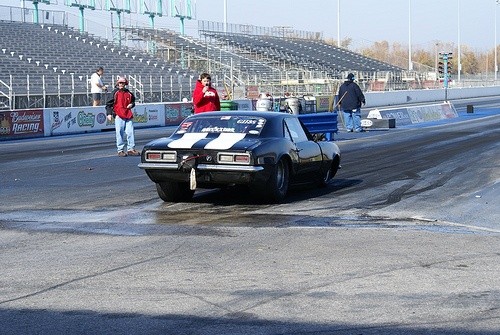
[0,21,408,110]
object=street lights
[494,0,500,81]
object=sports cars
[136,111,344,205]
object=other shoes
[128,150,140,156]
[118,151,127,156]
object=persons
[192,73,220,114]
[91,67,107,106]
[338,73,366,132]
[106,77,139,156]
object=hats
[116,78,128,86]
[345,73,354,79]
